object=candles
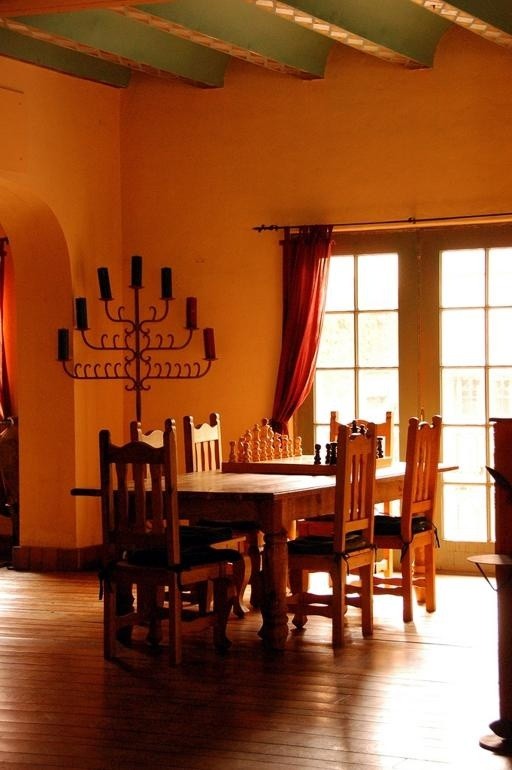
[56,255,216,363]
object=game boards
[222,455,392,475]
